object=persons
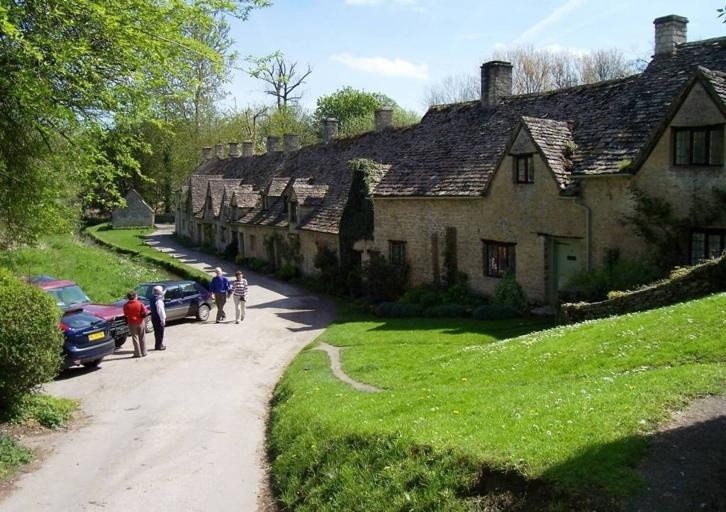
[229,270,249,324]
[150,285,167,350]
[208,267,232,323]
[123,291,148,358]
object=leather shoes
[156,346,165,350]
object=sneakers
[142,353,147,357]
[132,356,141,358]
[216,313,246,323]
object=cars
[31,279,212,376]
